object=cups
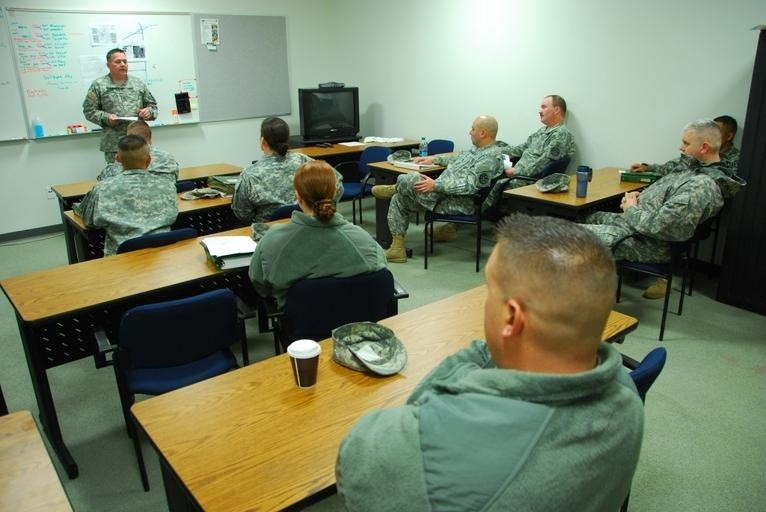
[287,339,321,389]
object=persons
[371,115,504,263]
[335,211,644,512]
[568,120,748,265]
[97,121,179,184]
[231,117,344,226]
[423,96,575,242]
[249,160,388,311]
[630,114,740,176]
[82,49,159,165]
[71,135,180,256]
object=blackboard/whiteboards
[0,7,200,142]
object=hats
[180,185,220,200]
[392,150,413,163]
[535,172,571,194]
[331,321,407,375]
[251,223,271,242]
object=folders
[620,171,662,183]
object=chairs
[271,204,302,221]
[619,346,666,512]
[258,267,409,357]
[117,228,198,254]
[111,289,250,492]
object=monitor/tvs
[298,87,360,143]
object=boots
[383,236,407,263]
[372,183,400,200]
[427,221,464,241]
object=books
[618,168,661,184]
[200,235,258,271]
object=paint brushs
[91,129,102,131]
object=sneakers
[644,277,668,298]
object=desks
[0,411,74,512]
[0,215,304,480]
[130,274,639,512]
[63,183,236,263]
[51,163,245,267]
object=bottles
[34,117,43,137]
[419,138,428,157]
[576,166,592,197]
